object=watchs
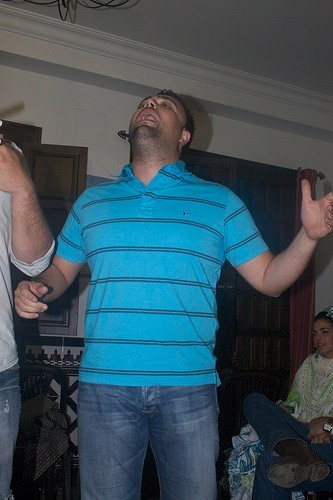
[323,423,333,433]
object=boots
[267,439,330,488]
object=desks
[23,364,82,500]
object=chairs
[209,373,282,473]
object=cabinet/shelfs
[179,150,315,428]
[0,119,88,336]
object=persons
[12,88,333,500]
[241,305,333,500]
[0,133,55,500]
